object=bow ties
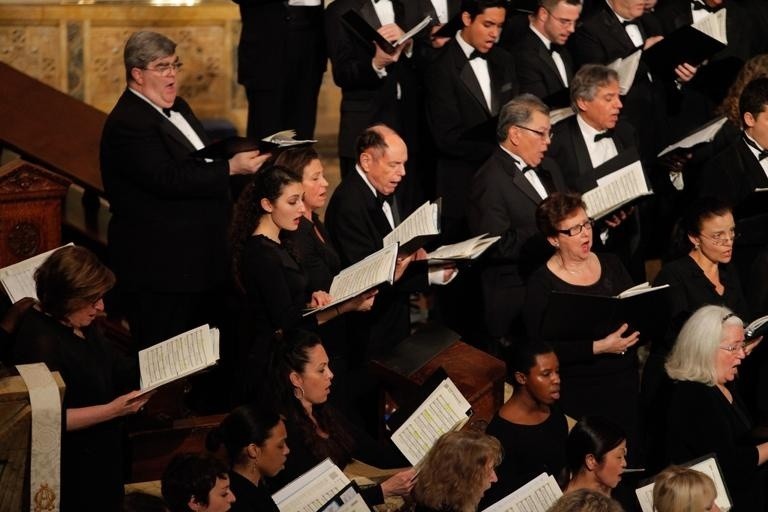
[376,194,393,206]
[548,11,583,28]
[621,19,643,27]
[594,131,613,140]
[467,47,489,62]
[523,165,531,174]
[758,149,768,161]
[549,42,564,54]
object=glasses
[140,65,183,77]
[516,125,554,140]
[720,343,746,352]
[558,219,595,237]
[701,232,735,245]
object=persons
[162,0,768,511]
[100,31,273,349]
[0,245,158,511]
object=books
[128,323,221,398]
[1,239,79,305]
